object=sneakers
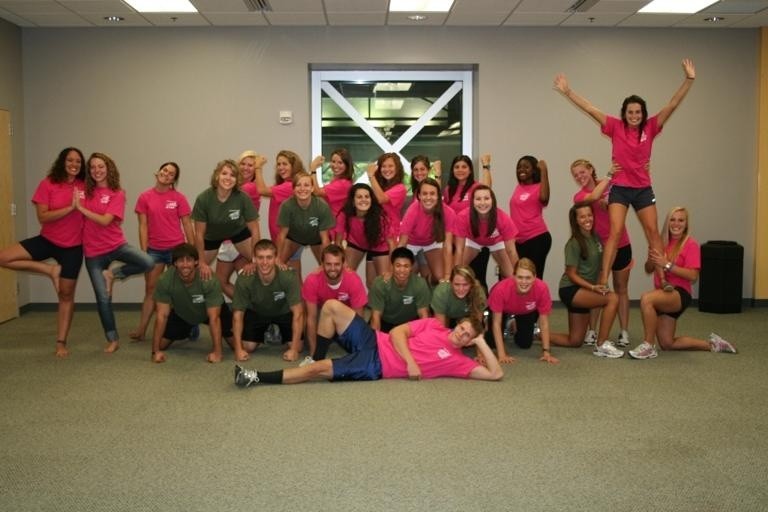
[234,365,257,385]
[300,358,317,365]
[662,282,673,290]
[584,330,658,359]
[710,333,737,353]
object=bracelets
[592,284,595,290]
[605,171,613,179]
[664,262,673,271]
[687,76,695,80]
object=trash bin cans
[698,241,745,314]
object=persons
[254,149,559,364]
[76,152,156,353]
[191,159,260,281]
[571,159,634,347]
[532,202,625,359]
[552,59,696,291]
[153,243,235,363]
[216,150,259,301]
[629,207,737,360]
[1,148,87,357]
[234,299,503,388]
[233,239,305,362]
[129,162,195,342]
